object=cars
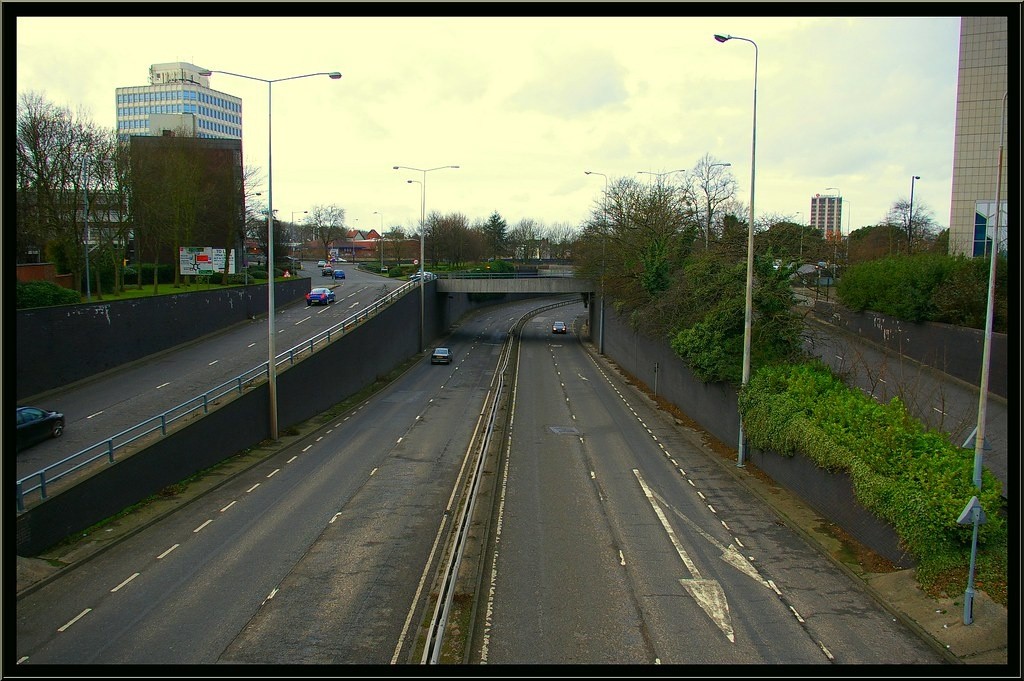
[552,321,566,333]
[305,288,336,307]
[317,255,346,280]
[409,271,436,279]
[16,406,63,453]
[288,257,300,264]
[431,348,453,364]
[773,260,837,285]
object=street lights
[374,166,460,351]
[584,171,608,354]
[714,34,759,467]
[704,163,732,250]
[85,160,113,300]
[200,70,342,439]
[825,187,841,284]
[843,199,850,262]
[638,169,686,200]
[907,175,920,253]
[351,218,358,264]
[292,210,307,264]
